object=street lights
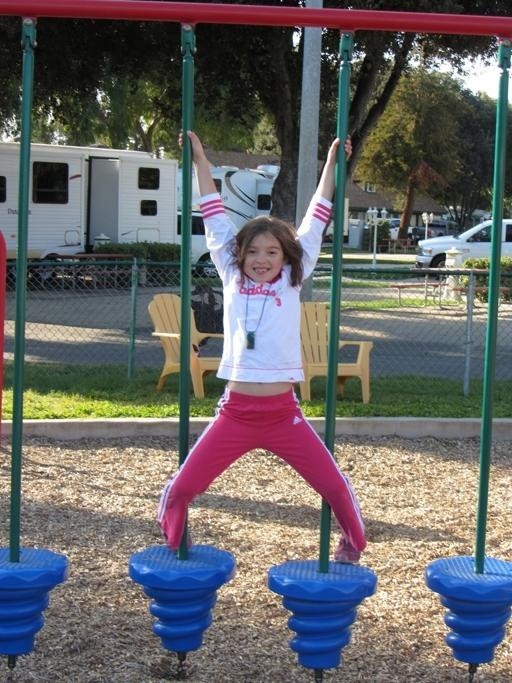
[367,205,387,275]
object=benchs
[386,280,511,308]
[35,266,146,289]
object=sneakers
[334,535,361,565]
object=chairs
[148,291,375,404]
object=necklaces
[243,279,270,349]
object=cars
[363,215,461,242]
[416,219,512,269]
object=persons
[158,130,369,563]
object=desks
[408,266,489,309]
[49,252,132,289]
